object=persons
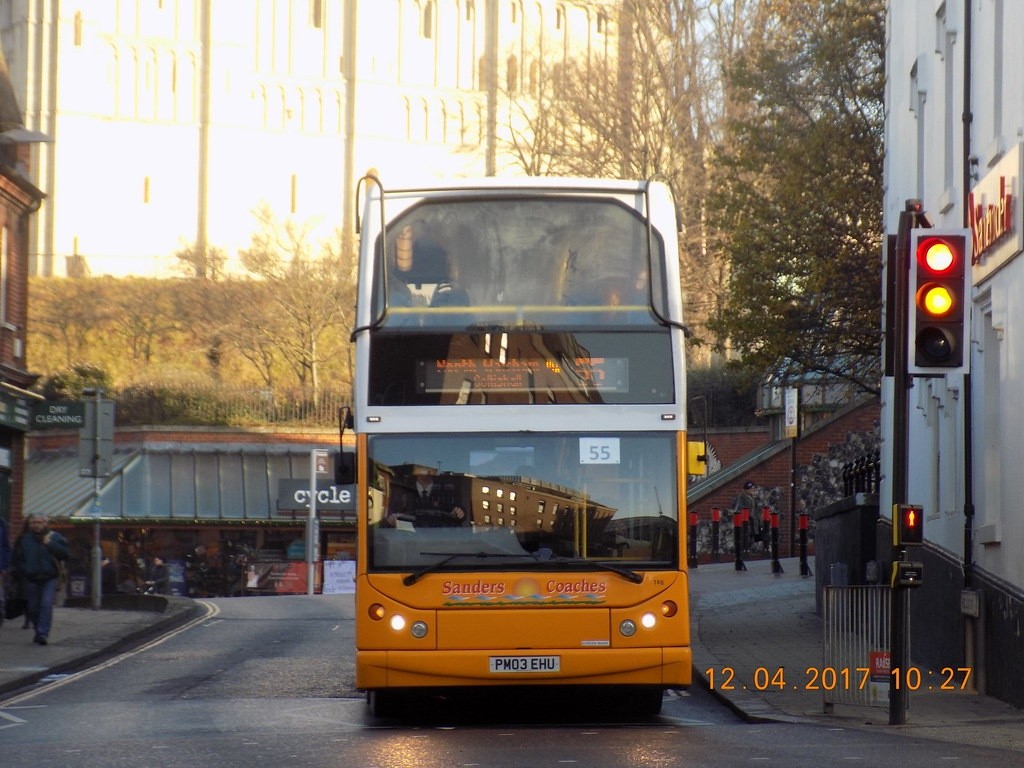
[731,481,756,517]
[137,557,169,596]
[0,515,11,579]
[23,513,71,646]
[384,467,463,527]
[13,514,36,628]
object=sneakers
[34,636,47,644]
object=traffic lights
[908,226,972,377]
[893,503,924,547]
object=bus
[344,170,695,723]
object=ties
[422,490,428,503]
